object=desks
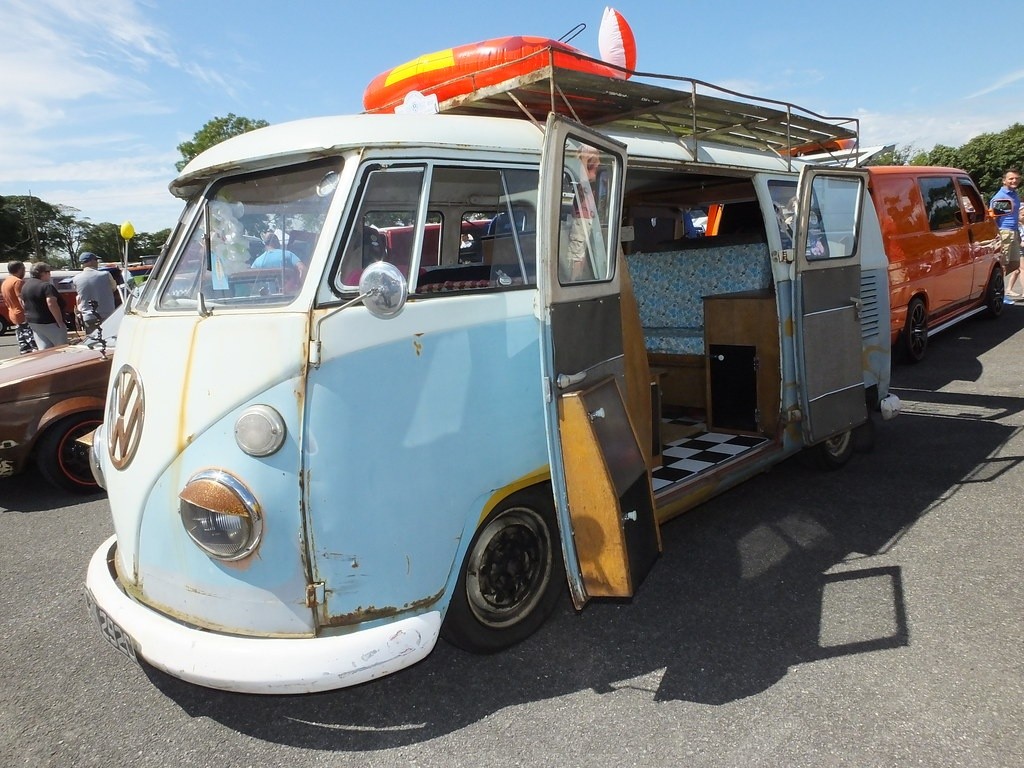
[700,288,780,440]
[650,368,666,467]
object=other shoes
[1002,296,1015,305]
[1005,290,1021,297]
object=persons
[928,188,957,223]
[380,273,393,308]
[73,252,116,335]
[21,262,67,351]
[989,169,1024,298]
[567,144,599,281]
[461,234,472,248]
[251,233,307,293]
[2,261,37,354]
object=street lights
[119,221,135,304]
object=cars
[0,260,201,337]
[688,211,709,235]
[0,265,302,494]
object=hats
[79,252,102,264]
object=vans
[287,218,490,270]
[864,164,1014,364]
[83,48,890,693]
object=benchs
[621,242,775,356]
